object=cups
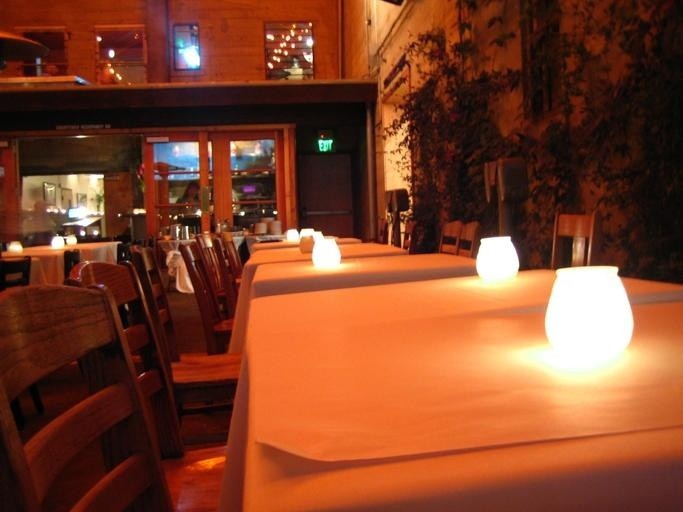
[169,224,191,240]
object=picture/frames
[39,181,89,211]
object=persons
[177,179,199,213]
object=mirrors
[261,18,315,81]
[171,20,201,71]
[94,23,149,85]
[19,24,68,79]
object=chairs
[387,211,483,257]
[2,225,251,512]
[548,212,605,266]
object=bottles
[475,236,521,284]
[50,235,78,250]
[544,264,634,365]
[7,241,24,254]
[285,226,342,271]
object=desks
[224,235,683,512]
